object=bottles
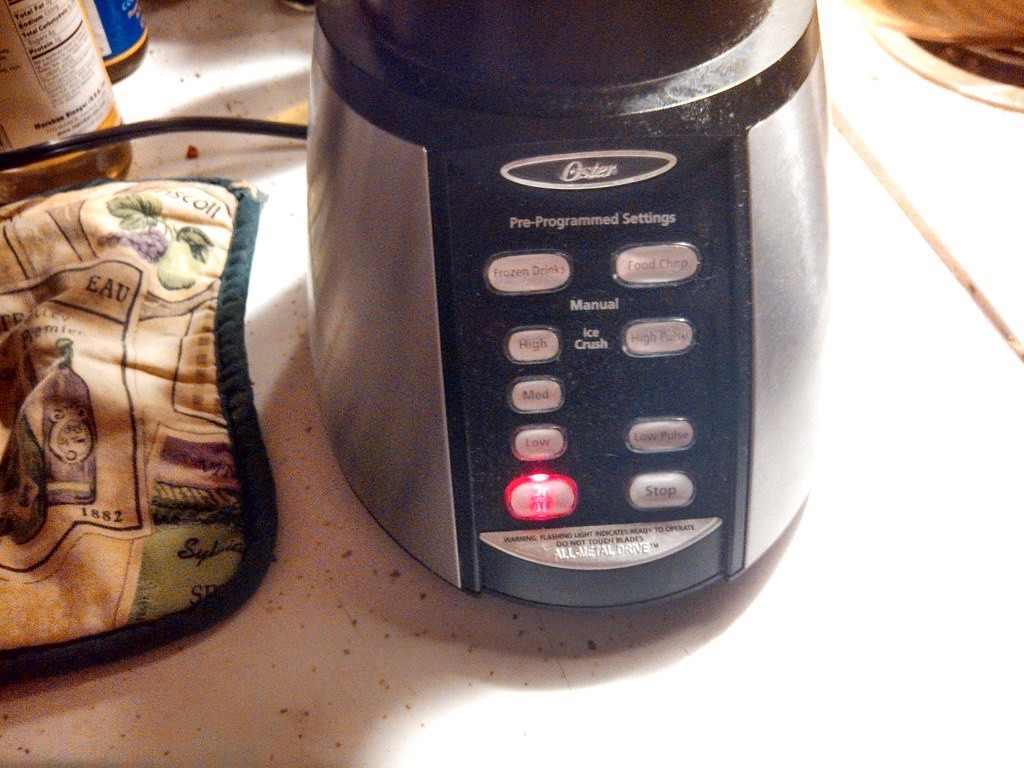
[84,0,148,83]
[0,0,133,204]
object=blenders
[306,0,836,609]
